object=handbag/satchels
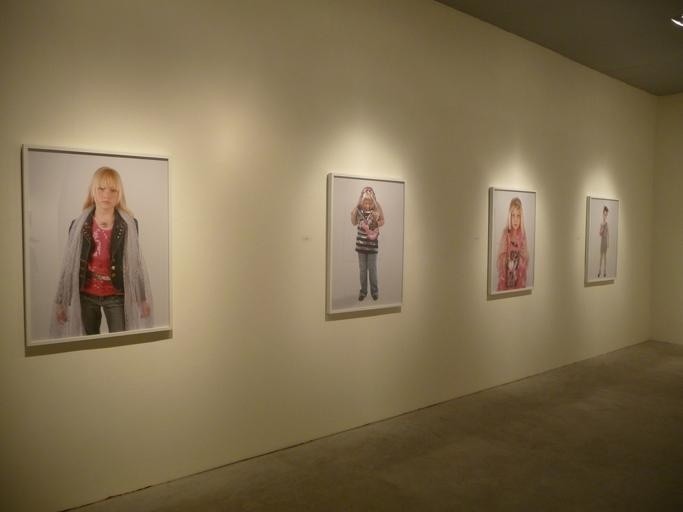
[357,207,379,240]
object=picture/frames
[584,195,619,286]
[487,187,536,300]
[326,172,405,319]
[22,144,174,350]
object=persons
[348,184,387,301]
[595,205,609,279]
[492,196,530,292]
[50,165,151,340]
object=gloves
[369,220,378,230]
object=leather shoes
[358,296,365,300]
[373,295,377,300]
